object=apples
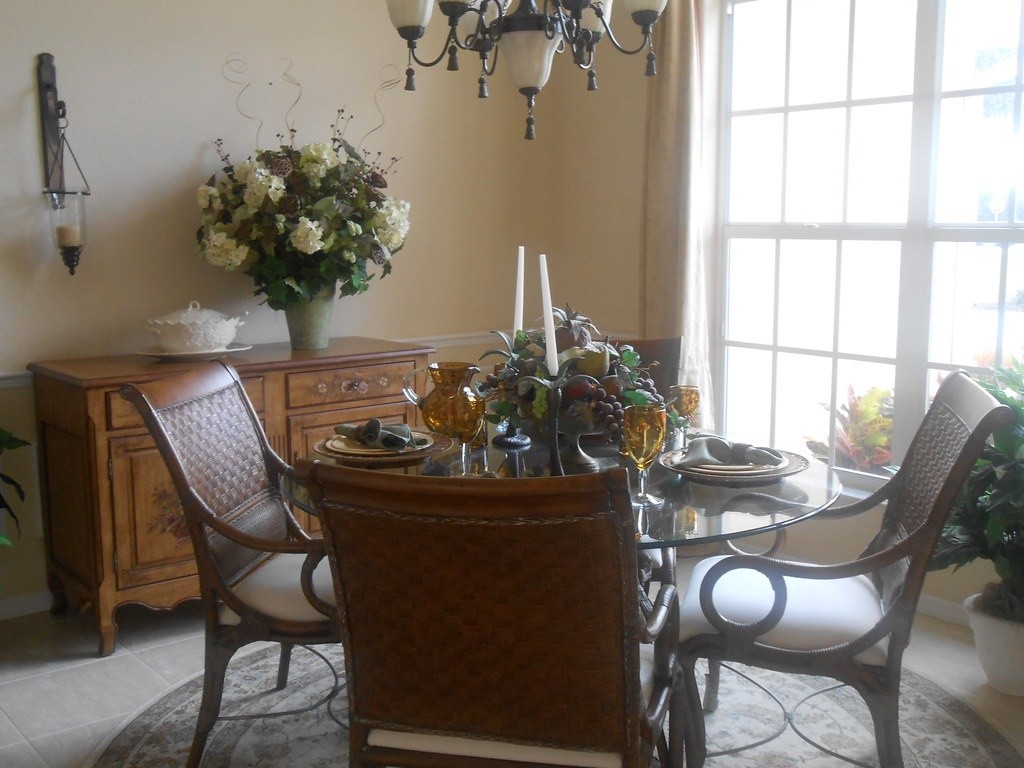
[562,374,601,405]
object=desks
[302,436,843,582]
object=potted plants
[931,359,1024,698]
[807,383,893,493]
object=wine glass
[493,362,522,432]
[668,385,700,447]
[623,405,666,509]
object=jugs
[402,361,480,438]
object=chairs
[674,370,1007,768]
[294,455,696,767]
[121,358,342,768]
[609,332,685,406]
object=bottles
[446,385,488,448]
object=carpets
[84,652,1023,768]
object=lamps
[385,0,668,144]
[42,102,91,274]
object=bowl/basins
[143,310,248,353]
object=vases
[497,424,607,477]
[284,270,335,348]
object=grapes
[532,366,550,419]
[479,372,503,391]
[592,377,666,436]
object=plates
[659,447,809,487]
[312,429,455,464]
[135,343,255,362]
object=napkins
[333,423,413,451]
[669,432,782,474]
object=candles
[540,253,559,379]
[510,244,525,342]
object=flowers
[188,112,411,307]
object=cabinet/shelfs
[24,334,437,658]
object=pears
[578,334,609,378]
